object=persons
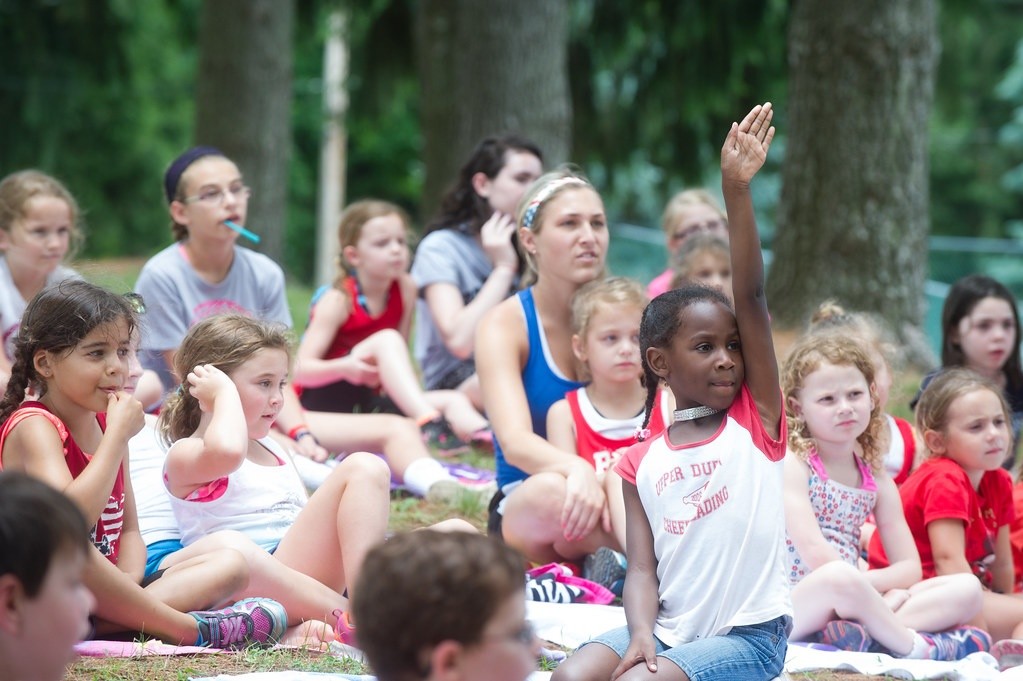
[129,143,497,515]
[409,133,547,418]
[549,102,795,681]
[289,199,496,458]
[0,282,348,681]
[353,530,543,681]
[780,271,1023,672]
[476,165,740,566]
[152,318,491,612]
[1,168,95,401]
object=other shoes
[425,477,498,513]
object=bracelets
[288,425,310,440]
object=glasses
[418,620,533,675]
[183,183,251,208]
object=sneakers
[582,546,628,599]
[326,608,368,664]
[918,624,993,662]
[187,597,287,651]
[816,619,885,654]
[470,422,494,454]
[990,639,1023,672]
